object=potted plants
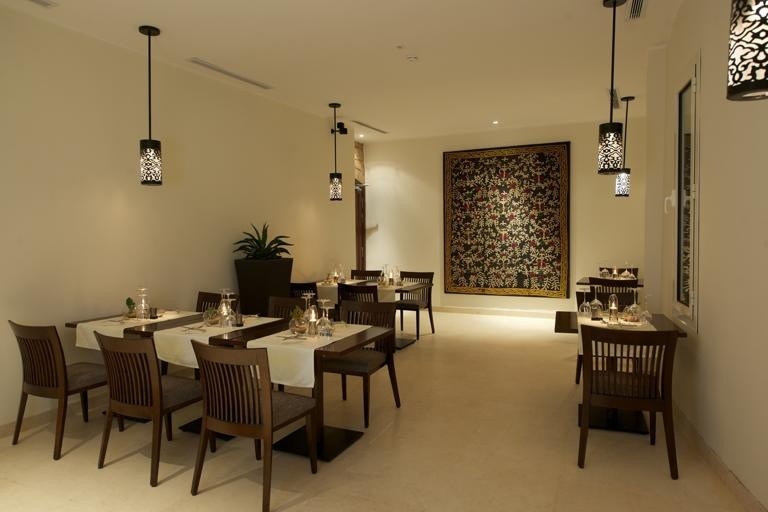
[234,223,293,316]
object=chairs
[325,297,404,428]
[93,329,202,486]
[588,276,639,295]
[397,271,438,340]
[196,292,236,313]
[5,318,107,459]
[269,297,315,321]
[577,324,679,479]
[350,270,381,279]
[289,281,318,298]
[337,282,378,303]
[189,341,319,512]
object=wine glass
[135,288,151,320]
[641,293,652,325]
[576,285,590,316]
[316,299,332,332]
[590,285,601,314]
[218,288,236,326]
[629,263,635,279]
[624,263,629,277]
[601,264,610,277]
[612,264,618,273]
[629,287,641,319]
[301,296,312,322]
[321,306,335,338]
[302,293,315,320]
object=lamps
[727,1,768,101]
[136,25,163,185]
[615,97,633,197]
[327,102,344,200]
[597,1,626,175]
